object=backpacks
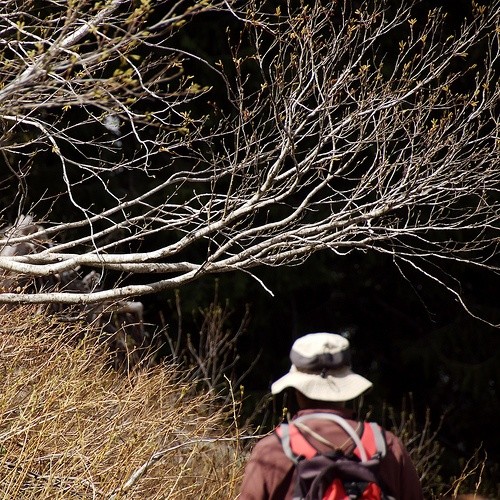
[276,420,393,499]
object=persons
[240,332,427,500]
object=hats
[269,331,374,403]
